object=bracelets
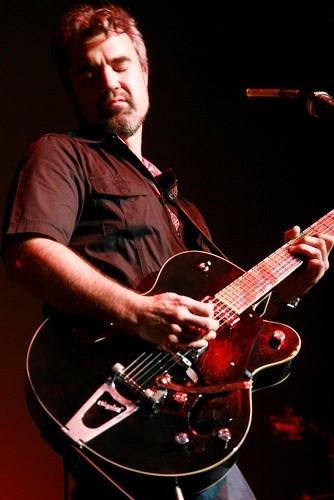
[271,287,301,311]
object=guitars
[25,209,334,500]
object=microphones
[306,91,334,118]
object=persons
[4,5,334,500]
